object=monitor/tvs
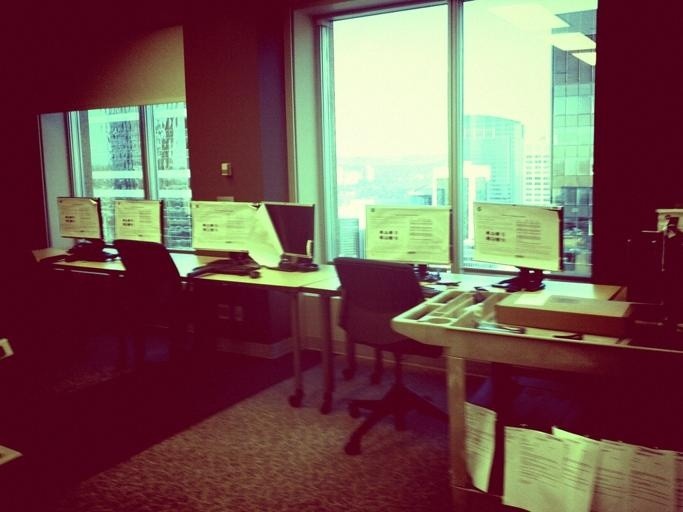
[56,197,103,244]
[472,202,563,291]
[190,201,261,265]
[366,205,453,283]
[260,202,317,272]
[115,199,164,244]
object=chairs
[332,258,448,456]
[110,238,215,290]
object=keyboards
[192,265,256,276]
[420,285,442,298]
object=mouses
[249,269,259,279]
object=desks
[389,289,682,512]
